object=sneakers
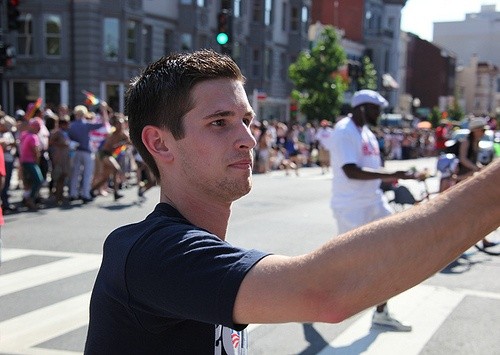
[371,311,412,331]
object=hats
[351,89,389,111]
[468,118,491,130]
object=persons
[329,90,415,332]
[84,48,500,355]
[0,101,158,214]
[249,119,336,174]
[370,126,435,161]
[435,112,500,249]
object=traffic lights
[217,8,234,56]
